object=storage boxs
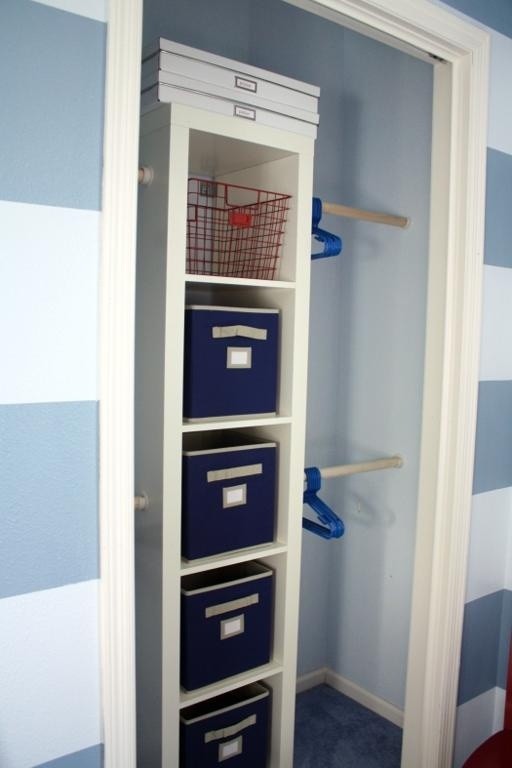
[186,302,283,420]
[181,561,276,696]
[180,684,274,767]
[182,430,281,567]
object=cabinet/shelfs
[134,103,320,767]
